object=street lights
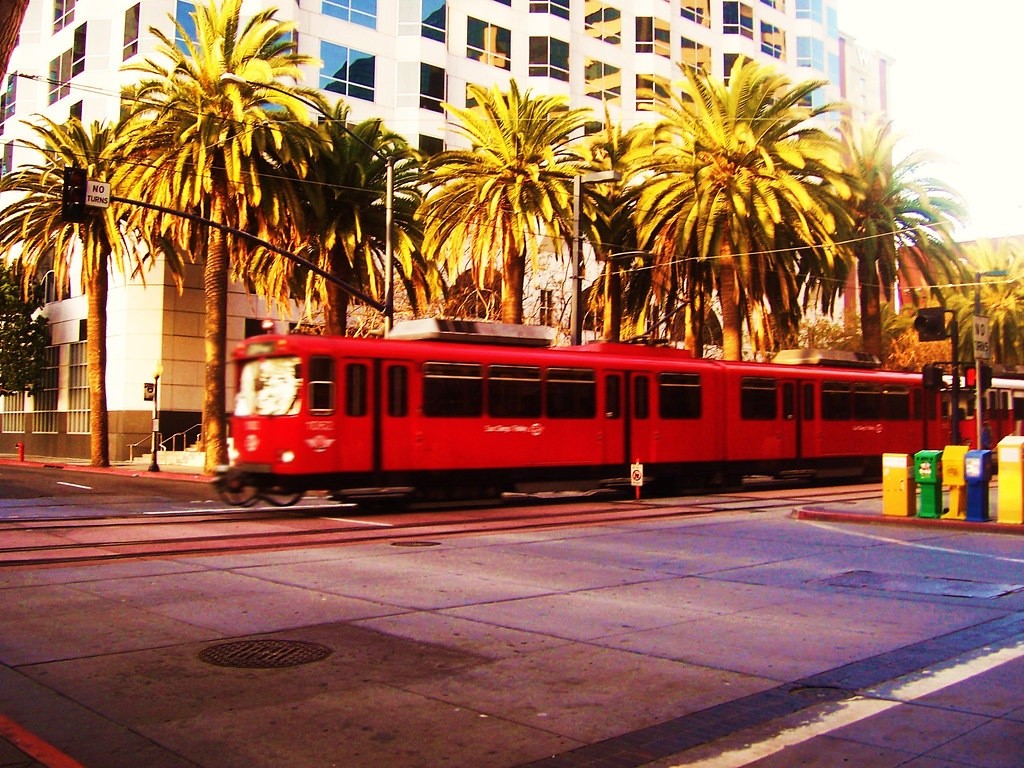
[221,73,395,340]
[973,270,1007,451]
[148,365,164,472]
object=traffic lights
[964,365,977,388]
[913,307,947,342]
[62,166,87,223]
[922,363,942,391]
[981,363,992,394]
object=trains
[212,315,1024,513]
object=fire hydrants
[15,442,24,462]
